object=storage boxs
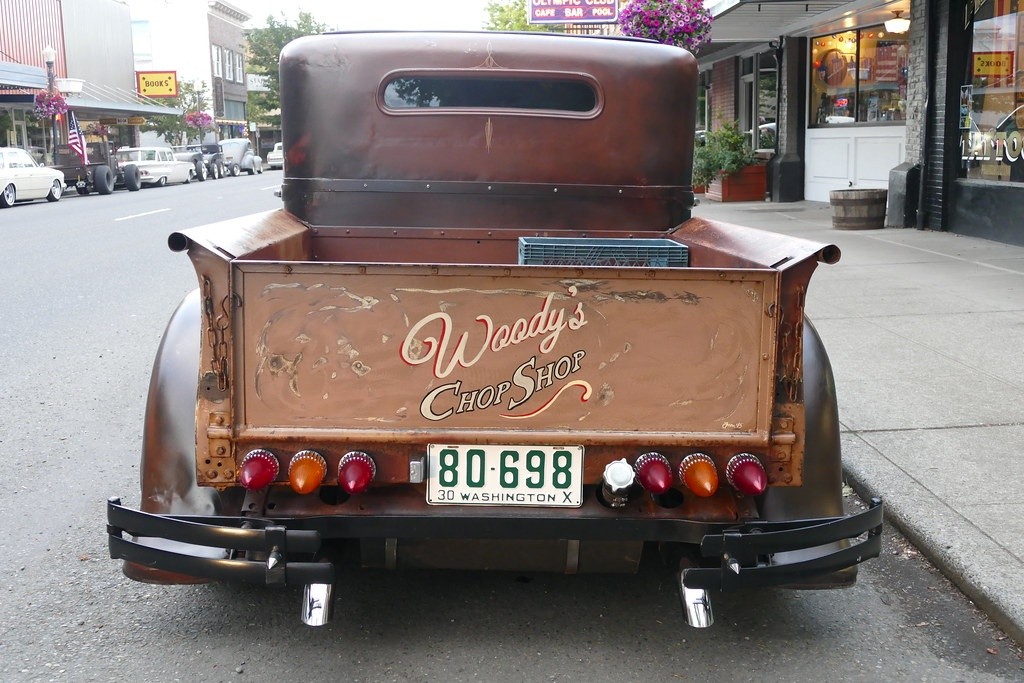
[518,236,688,267]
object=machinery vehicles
[174,143,241,182]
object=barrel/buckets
[830,189,888,232]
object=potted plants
[700,119,765,201]
[691,147,715,192]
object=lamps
[885,10,911,34]
[42,44,55,68]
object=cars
[217,138,263,175]
[267,141,284,171]
[0,146,67,208]
[115,146,197,187]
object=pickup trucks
[103,32,888,628]
[49,140,141,194]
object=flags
[66,108,90,167]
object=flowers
[620,0,712,54]
